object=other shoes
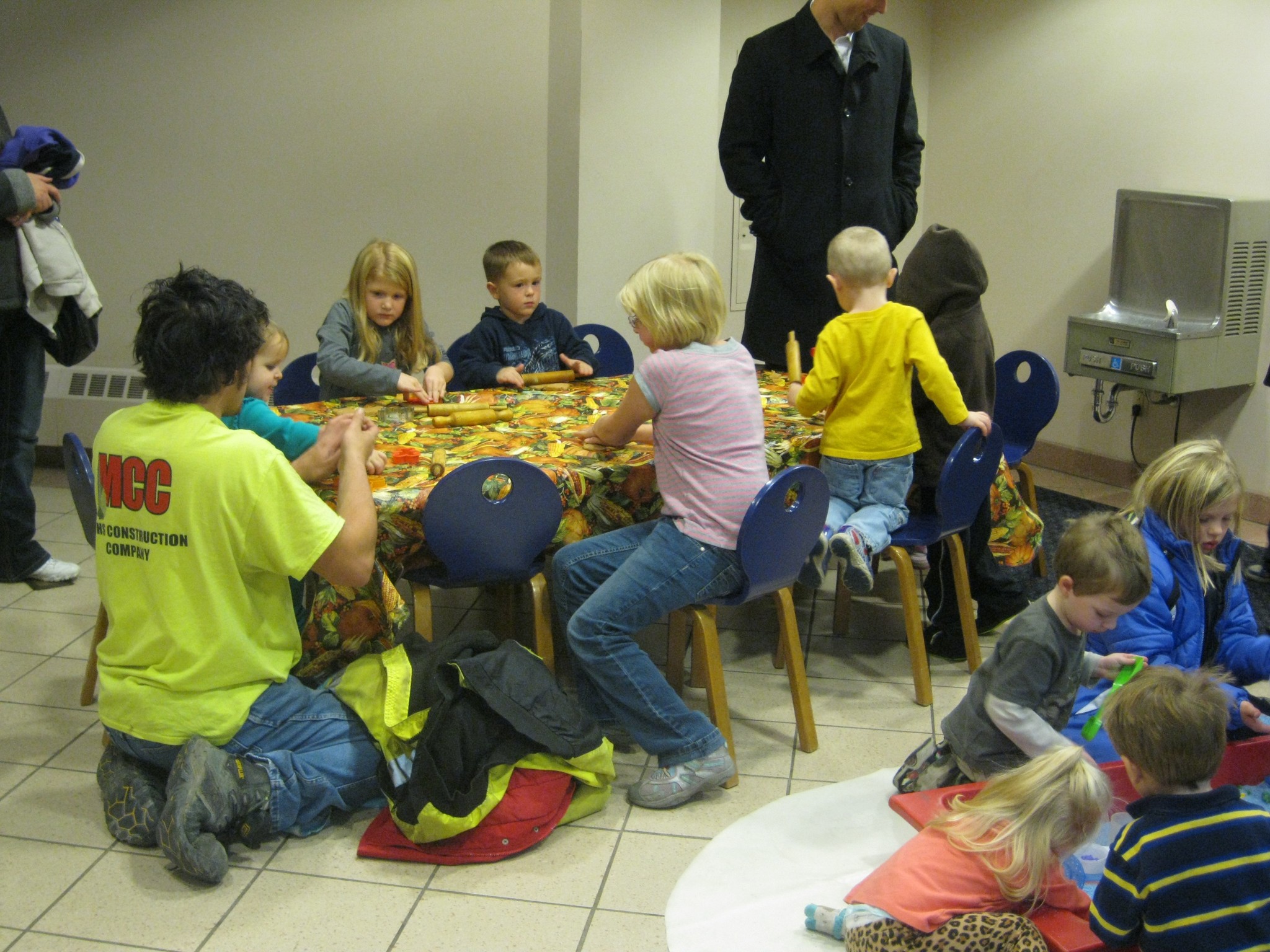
[907,628,965,661]
[976,599,1030,634]
[597,720,640,754]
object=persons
[787,227,991,594]
[893,222,1013,662]
[445,240,599,391]
[92,262,389,883]
[548,252,770,809]
[317,238,453,405]
[893,511,1147,795]
[843,744,1112,952]
[719,0,925,372]
[218,320,387,627]
[1059,439,1270,767]
[0,105,80,584]
[1090,665,1270,952]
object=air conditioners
[38,364,155,450]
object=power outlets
[1132,392,1151,417]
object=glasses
[628,315,640,327]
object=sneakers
[627,746,736,807]
[152,734,272,884]
[830,525,875,592]
[97,744,167,848]
[893,738,951,792]
[797,524,834,589]
[28,555,79,581]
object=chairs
[989,350,1061,579]
[664,465,832,789]
[572,323,635,378]
[773,422,1001,708]
[63,433,110,703]
[273,353,321,407]
[407,453,556,671]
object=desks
[269,370,1043,679]
